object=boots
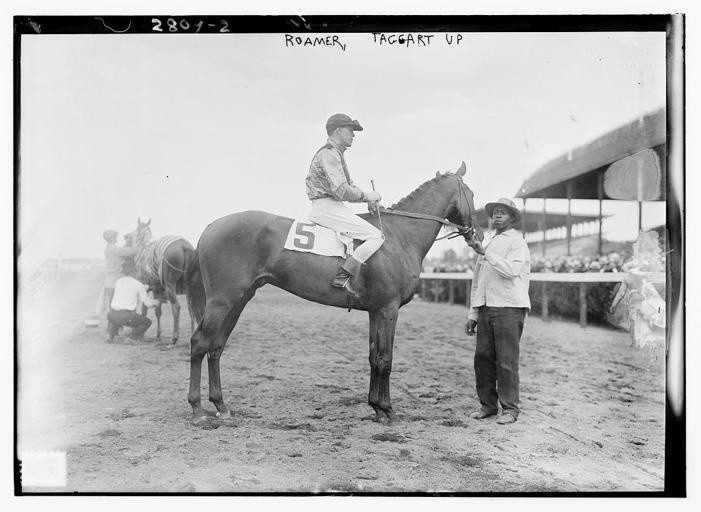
[332,256,362,298]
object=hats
[103,229,119,241]
[486,197,522,223]
[326,113,364,133]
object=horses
[128,216,196,351]
[180,158,485,432]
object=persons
[97,229,136,338]
[422,247,666,273]
[609,260,666,348]
[304,112,386,297]
[464,196,533,425]
[104,261,160,343]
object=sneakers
[470,409,489,419]
[106,334,116,344]
[496,413,517,424]
[124,336,141,345]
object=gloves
[363,191,382,202]
